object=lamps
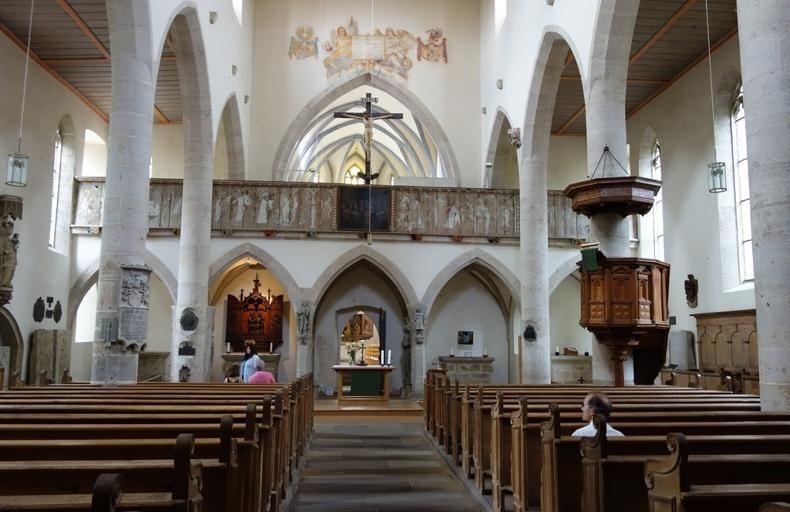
[702,0,729,195]
[227,342,230,352]
[350,0,386,61]
[269,342,273,353]
[4,0,34,187]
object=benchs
[421,369,790,511]
[0,366,316,512]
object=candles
[483,347,488,355]
[450,348,453,355]
[380,349,392,365]
[556,346,559,352]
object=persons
[298,300,311,334]
[248,358,276,384]
[342,110,394,161]
[238,343,266,384]
[324,26,362,72]
[413,307,425,335]
[256,311,266,333]
[383,27,408,72]
[247,312,257,333]
[571,391,626,437]
[223,364,243,383]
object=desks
[331,363,396,405]
